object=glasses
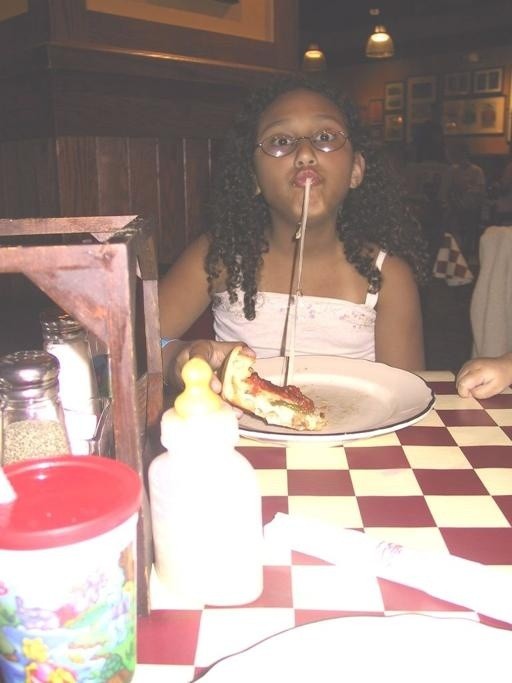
[250,127,352,158]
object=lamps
[368,0,390,44]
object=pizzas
[219,178,317,432]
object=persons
[158,86,425,395]
[455,350,512,401]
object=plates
[223,355,437,443]
[190,615,512,683]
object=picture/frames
[367,63,507,147]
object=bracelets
[160,335,182,346]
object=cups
[1,455,143,683]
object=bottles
[1,303,103,468]
[146,356,265,608]
